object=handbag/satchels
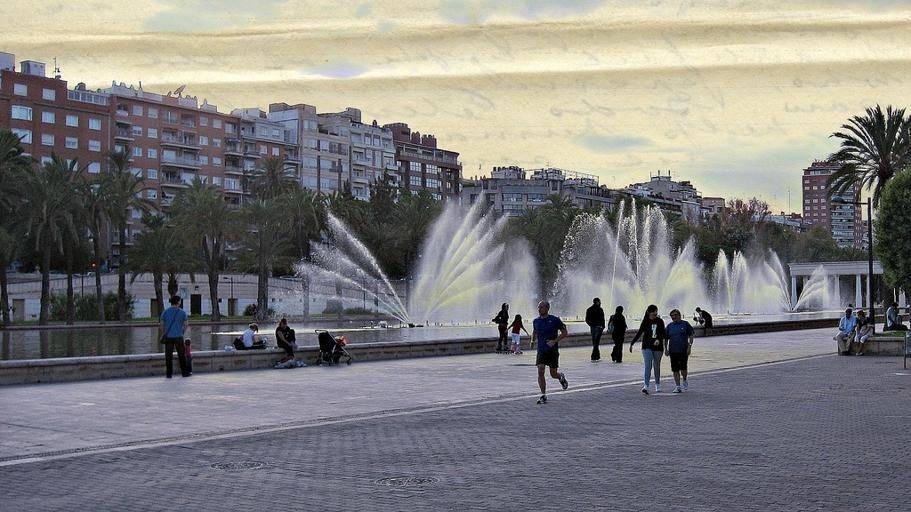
[860,324,874,336]
[159,333,168,344]
[233,337,246,350]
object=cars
[73,271,95,278]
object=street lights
[222,276,234,316]
[832,197,877,332]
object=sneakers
[559,372,568,390]
[655,384,661,392]
[673,381,689,393]
[642,384,650,394]
[537,395,548,404]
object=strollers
[314,328,354,366]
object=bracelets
[531,340,535,344]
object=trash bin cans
[228,298,238,315]
[190,294,201,315]
[151,298,158,317]
[180,288,187,299]
[13,299,24,324]
[195,286,199,294]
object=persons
[607,305,629,362]
[585,297,606,362]
[184,338,193,374]
[837,308,858,355]
[696,307,714,336]
[693,317,706,328]
[507,315,530,353]
[630,304,667,396]
[855,310,875,355]
[665,309,695,393]
[159,295,190,378]
[529,300,569,404]
[234,322,268,350]
[493,302,510,350]
[276,319,297,361]
[887,302,907,330]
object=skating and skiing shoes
[511,345,524,355]
[495,344,510,355]
